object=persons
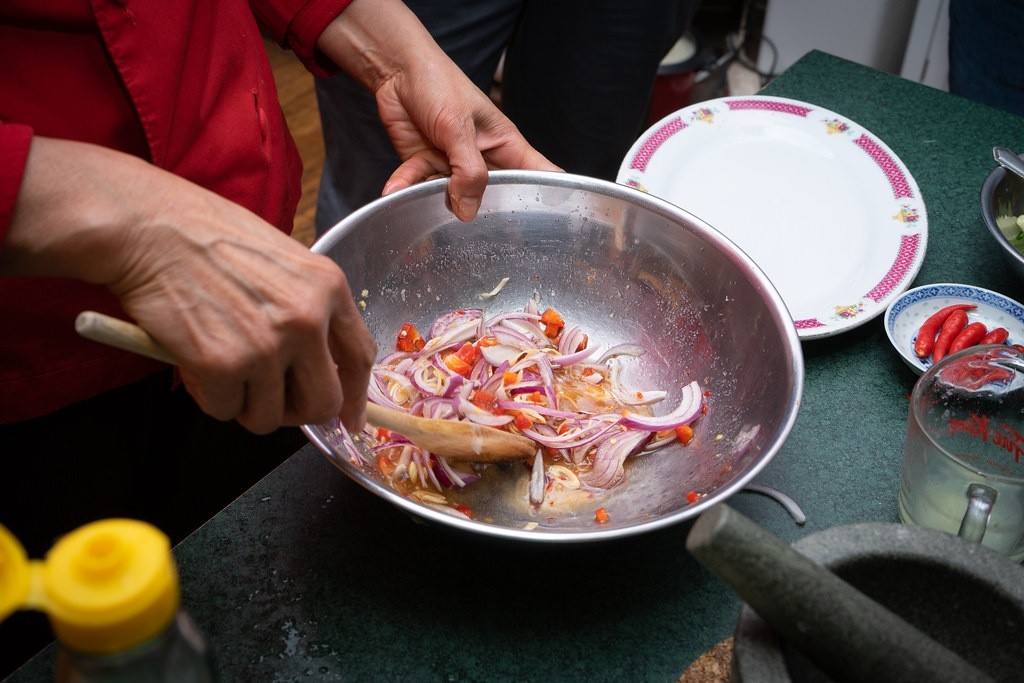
[304,2,695,252]
[0,0,572,681]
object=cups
[899,342,1024,569]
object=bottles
[0,516,223,683]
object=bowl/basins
[884,282,1024,400]
[298,170,805,545]
[732,521,1024,683]
[979,154,1024,276]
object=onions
[362,298,706,525]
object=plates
[613,95,930,339]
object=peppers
[915,301,1024,394]
